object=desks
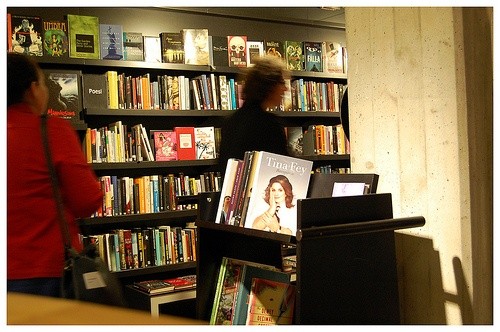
[124,275,196,317]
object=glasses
[276,80,286,86]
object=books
[215,151,313,236]
[7,13,348,295]
[210,256,296,326]
[331,182,370,197]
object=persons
[252,175,297,236]
[7,52,103,297]
[219,56,289,187]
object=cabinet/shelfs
[175,192,425,324]
[28,57,351,277]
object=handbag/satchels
[61,247,124,307]
